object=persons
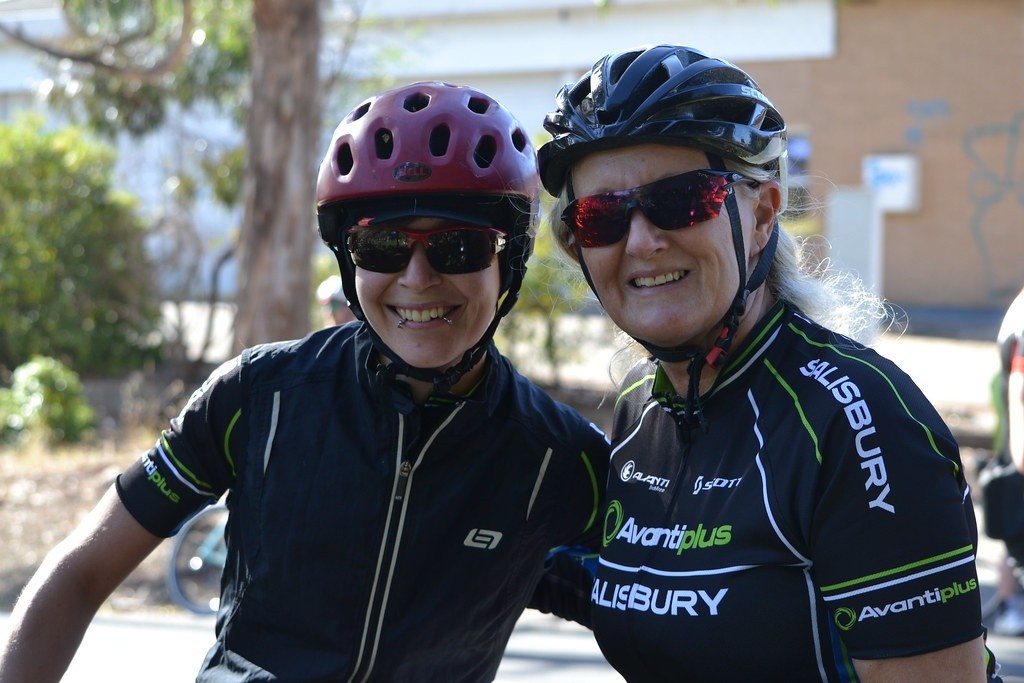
[315,276,356,327]
[0,80,613,683]
[526,45,1003,683]
[981,289,1024,638]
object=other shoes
[994,584,1024,636]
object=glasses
[347,218,509,274]
[561,169,760,248]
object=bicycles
[165,507,229,617]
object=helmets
[536,45,787,199]
[316,81,541,246]
